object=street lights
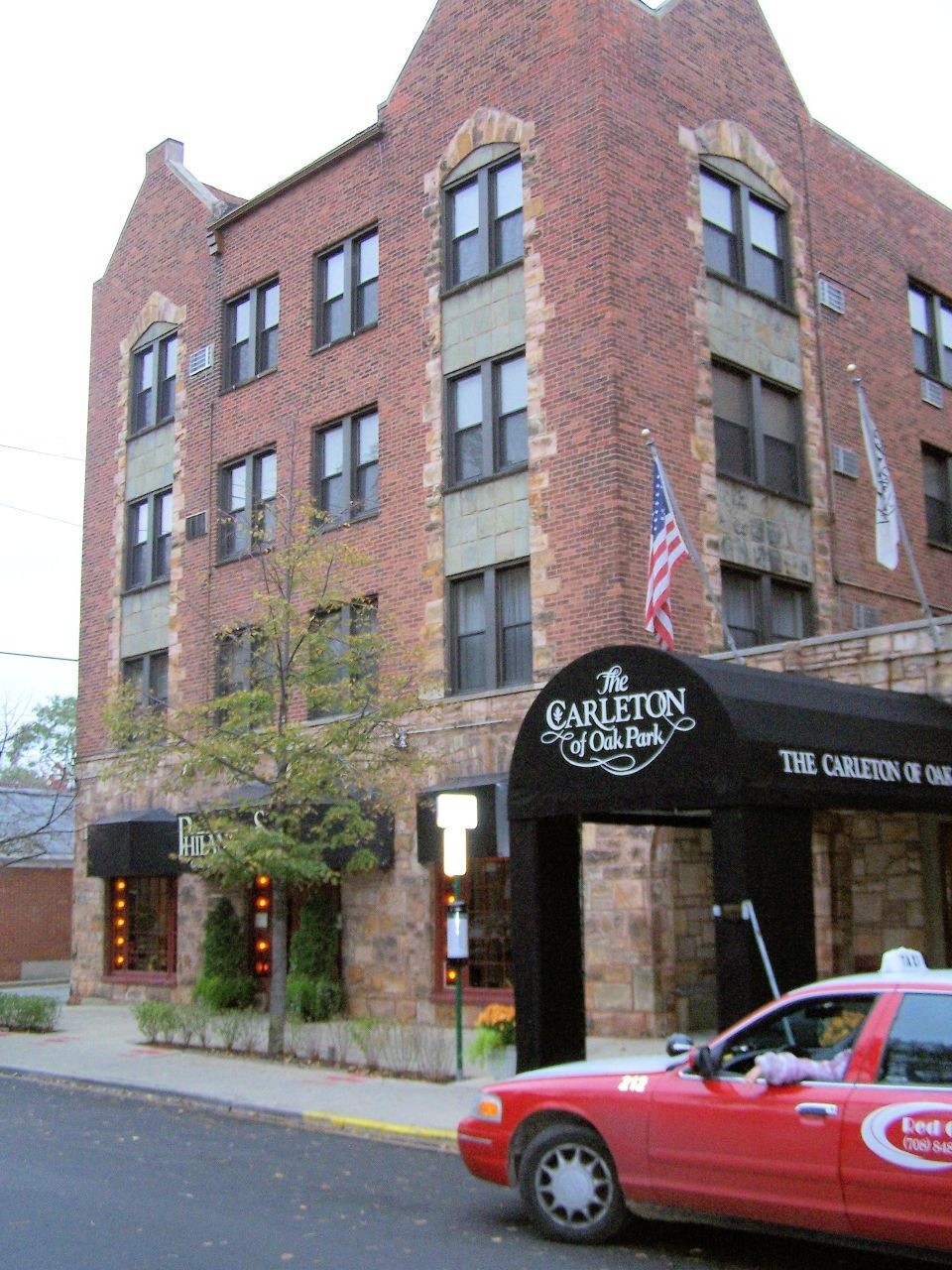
[435,793,481,1081]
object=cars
[458,943,951,1265]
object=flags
[852,379,898,570]
[644,457,690,651]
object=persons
[745,1051,851,1084]
